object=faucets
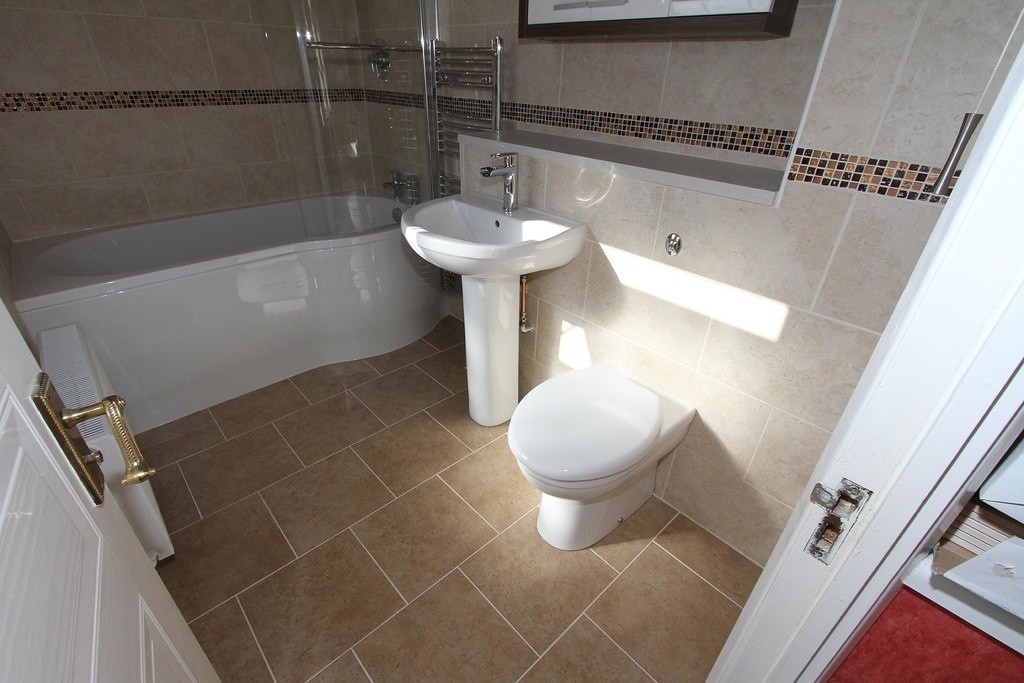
[480,152,519,212]
[383,169,418,191]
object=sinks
[401,194,587,276]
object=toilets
[508,353,699,552]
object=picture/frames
[518,0,798,39]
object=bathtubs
[15,195,440,434]
